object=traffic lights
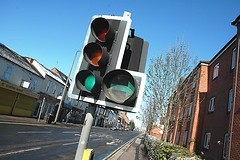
[75,16,117,97]
[100,68,138,106]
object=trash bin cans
[46,113,54,124]
